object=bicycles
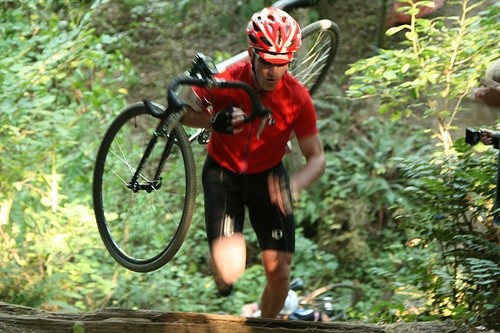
[92,0,342,272]
[290,278,358,321]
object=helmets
[246,6,302,64]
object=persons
[243,289,329,322]
[479,127,500,207]
[176,6,326,318]
